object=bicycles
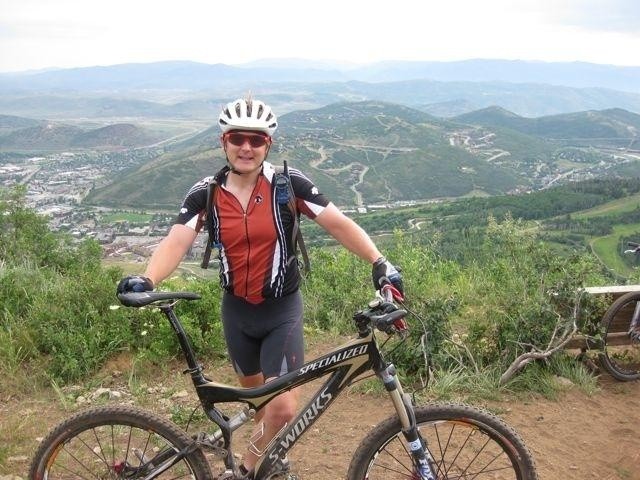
[28,275,536,480]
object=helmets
[218,98,278,136]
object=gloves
[117,275,154,293]
[372,256,406,299]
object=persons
[112,98,406,480]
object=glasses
[226,134,270,148]
